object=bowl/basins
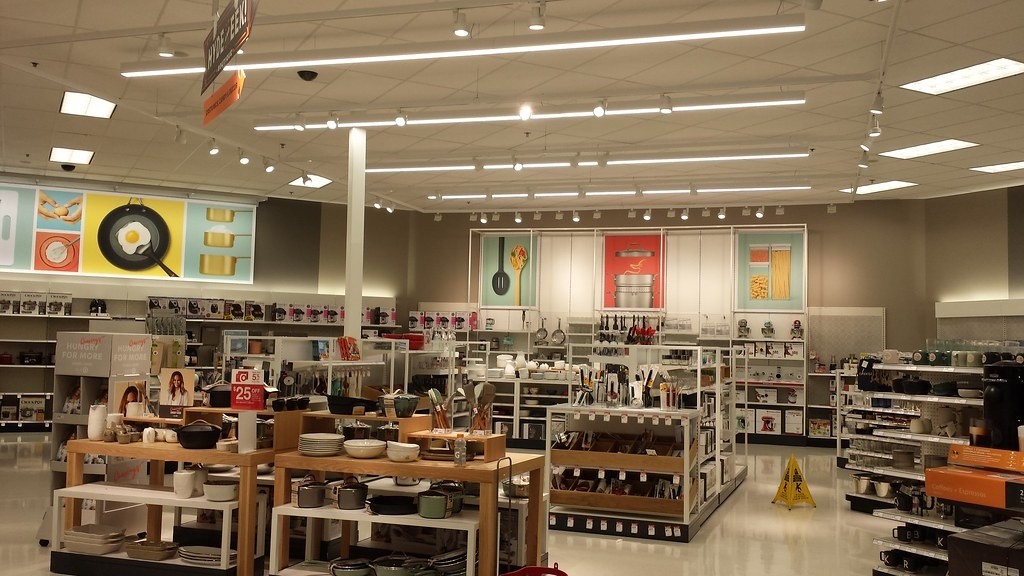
[529,387,557,395]
[202,480,239,501]
[545,371,558,380]
[343,439,420,462]
[531,373,544,380]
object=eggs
[118,222,151,254]
[54,206,69,216]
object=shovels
[613,313,618,330]
[600,315,604,330]
[492,237,510,295]
[510,245,527,306]
[605,314,609,331]
[434,389,450,429]
[620,315,627,334]
[457,381,496,434]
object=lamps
[528,0,546,30]
[157,32,174,57]
[453,7,469,37]
[179,98,886,226]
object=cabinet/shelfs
[0,312,1024,576]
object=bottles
[454,433,467,466]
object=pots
[0,299,71,316]
[172,419,222,449]
[536,317,566,344]
[98,198,180,278]
[199,207,253,275]
[322,388,420,447]
[0,349,43,365]
[201,380,231,408]
[291,472,530,576]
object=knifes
[428,381,494,435]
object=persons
[118,387,138,416]
[168,371,187,407]
[38,191,83,224]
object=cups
[104,402,179,444]
[847,337,1024,576]
[172,469,207,499]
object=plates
[519,399,538,417]
[64,523,237,566]
[298,433,345,456]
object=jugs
[87,405,106,441]
[496,350,569,380]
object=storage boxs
[755,409,782,434]
[551,421,565,440]
[948,444,1024,472]
[710,455,728,484]
[0,291,72,315]
[753,388,777,403]
[734,409,756,434]
[699,465,716,499]
[0,406,17,421]
[722,451,736,478]
[924,467,1024,513]
[19,397,45,421]
[785,410,804,434]
[785,343,804,358]
[147,296,478,331]
[767,343,785,358]
[808,419,830,437]
[755,342,766,357]
